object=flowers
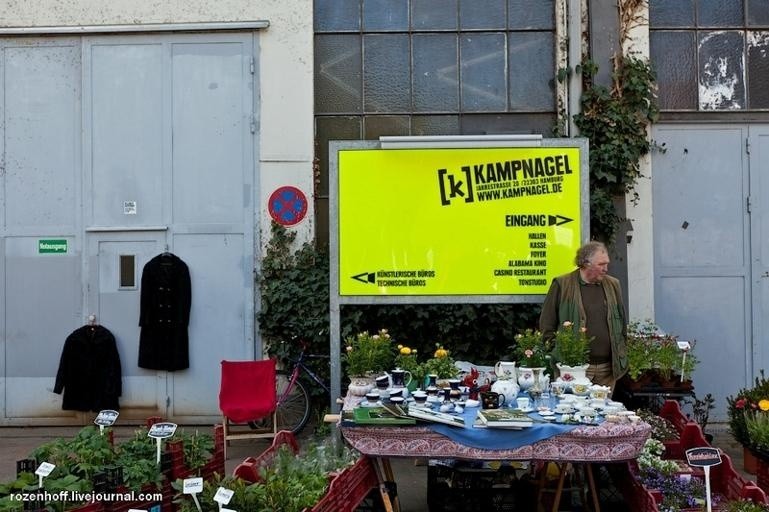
[344,328,462,379]
[743,399,769,458]
[512,328,553,367]
[553,320,596,366]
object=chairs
[219,357,281,459]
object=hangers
[86,315,98,333]
[160,243,173,266]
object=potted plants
[620,318,700,393]
[725,369,769,474]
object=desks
[326,370,652,512]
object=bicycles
[244,327,349,438]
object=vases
[556,362,593,392]
[757,459,769,496]
[518,367,546,390]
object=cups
[361,362,642,429]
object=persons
[539,242,630,401]
